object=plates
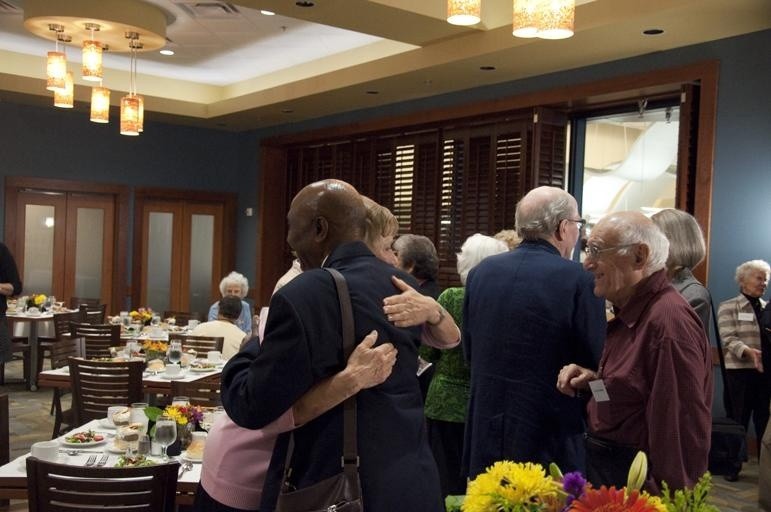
[6,311,18,315]
[190,366,215,372]
[62,436,106,447]
[180,452,203,463]
[160,374,184,380]
[105,439,136,453]
[99,418,117,430]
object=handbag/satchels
[275,464,364,512]
[707,416,744,477]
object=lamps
[445,1,576,41]
[45,21,146,136]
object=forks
[96,456,108,468]
[83,456,96,467]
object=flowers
[444,449,723,512]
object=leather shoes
[725,475,738,482]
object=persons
[1,237,22,388]
[390,186,711,499]
[718,259,771,483]
[207,268,256,338]
[194,294,246,366]
[190,195,462,512]
[269,247,305,307]
[221,176,445,511]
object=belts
[582,432,623,452]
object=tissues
[186,431,210,460]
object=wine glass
[44,295,62,313]
[155,415,177,466]
[169,340,182,363]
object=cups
[151,315,160,326]
[206,351,221,362]
[188,320,198,330]
[129,403,149,439]
[171,396,189,407]
[106,407,126,424]
[165,365,179,375]
[120,312,131,331]
[191,431,206,447]
[125,339,137,353]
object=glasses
[565,217,586,229]
[586,242,642,262]
